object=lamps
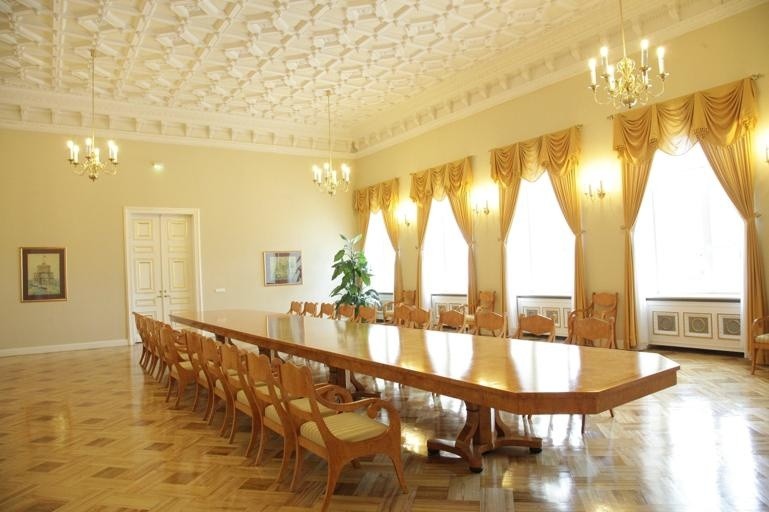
[64,47,122,182]
[308,88,355,197]
[582,1,672,115]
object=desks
[169,308,679,472]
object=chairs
[292,291,622,435]
[748,312,768,376]
[132,311,409,509]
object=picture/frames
[19,245,68,303]
[262,250,303,287]
